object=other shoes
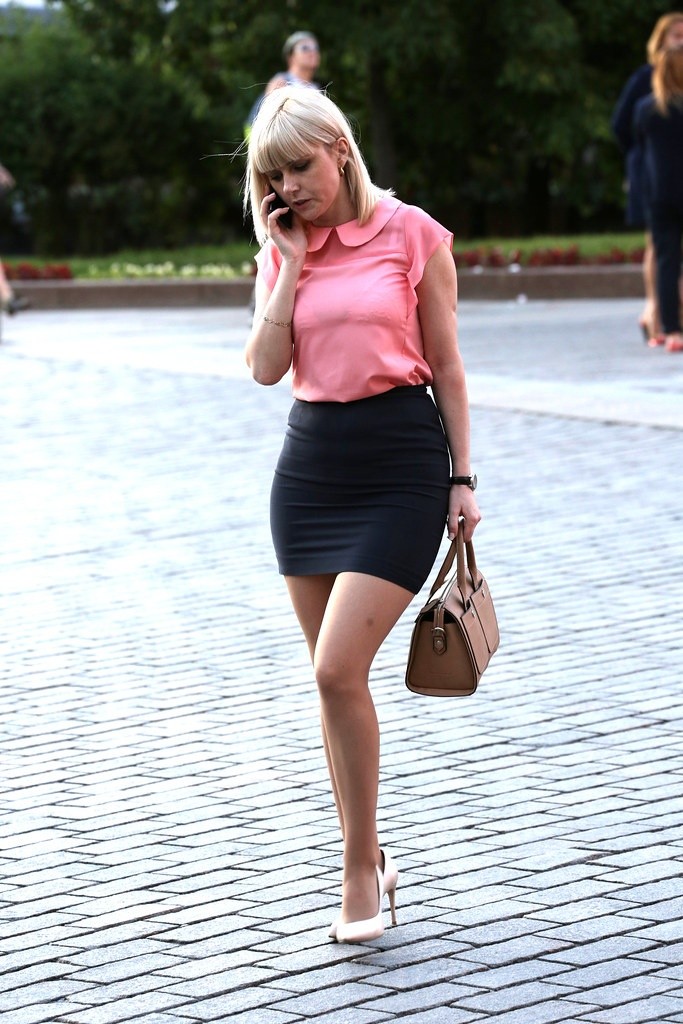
[639,318,664,345]
[665,331,683,351]
[4,295,30,315]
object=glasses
[294,45,319,53]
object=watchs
[450,473,477,491]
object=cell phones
[268,188,292,231]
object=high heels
[336,864,384,944]
[328,850,399,935]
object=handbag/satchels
[405,519,500,697]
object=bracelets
[260,316,292,329]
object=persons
[609,14,683,353]
[0,164,30,315]
[249,32,323,124]
[243,84,482,944]
